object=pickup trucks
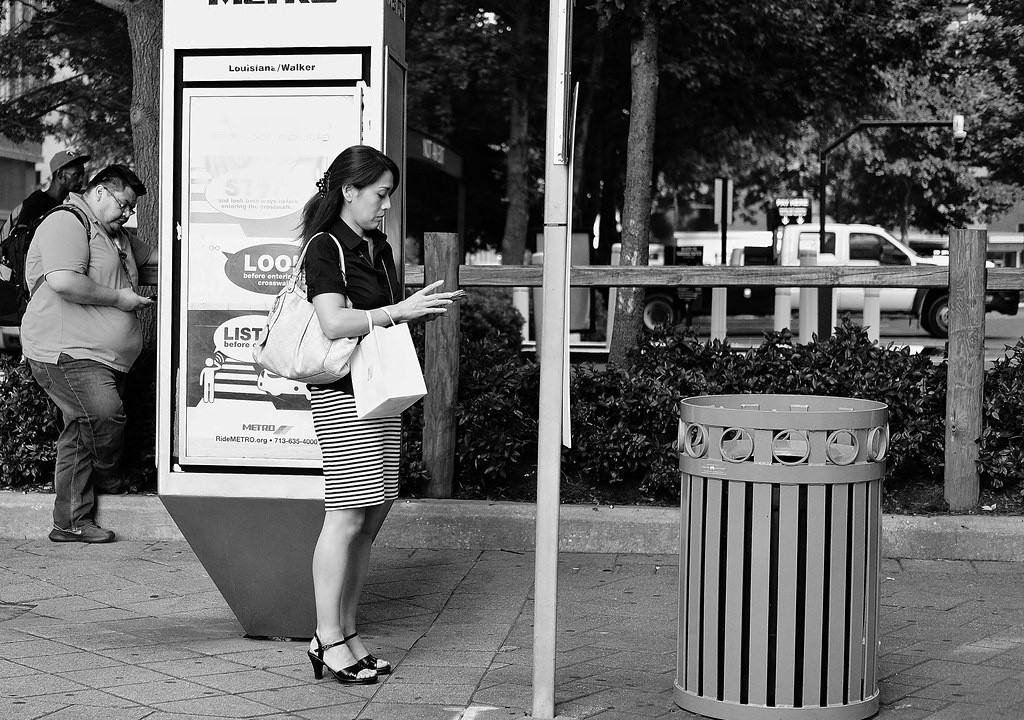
[632,225,1014,343]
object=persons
[298,144,455,687]
[649,196,675,264]
[13,150,85,364]
[20,164,159,544]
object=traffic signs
[772,196,813,226]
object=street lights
[818,116,966,255]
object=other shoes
[49,523,115,543]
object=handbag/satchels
[252,232,359,383]
[350,307,427,419]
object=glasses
[103,186,135,216]
[62,171,84,179]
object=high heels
[307,630,378,685]
[327,632,391,675]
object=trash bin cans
[671,394,890,720]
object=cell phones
[146,294,158,301]
[452,290,465,297]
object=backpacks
[0,204,91,352]
[0,191,47,244]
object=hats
[49,150,90,174]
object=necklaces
[380,256,394,305]
